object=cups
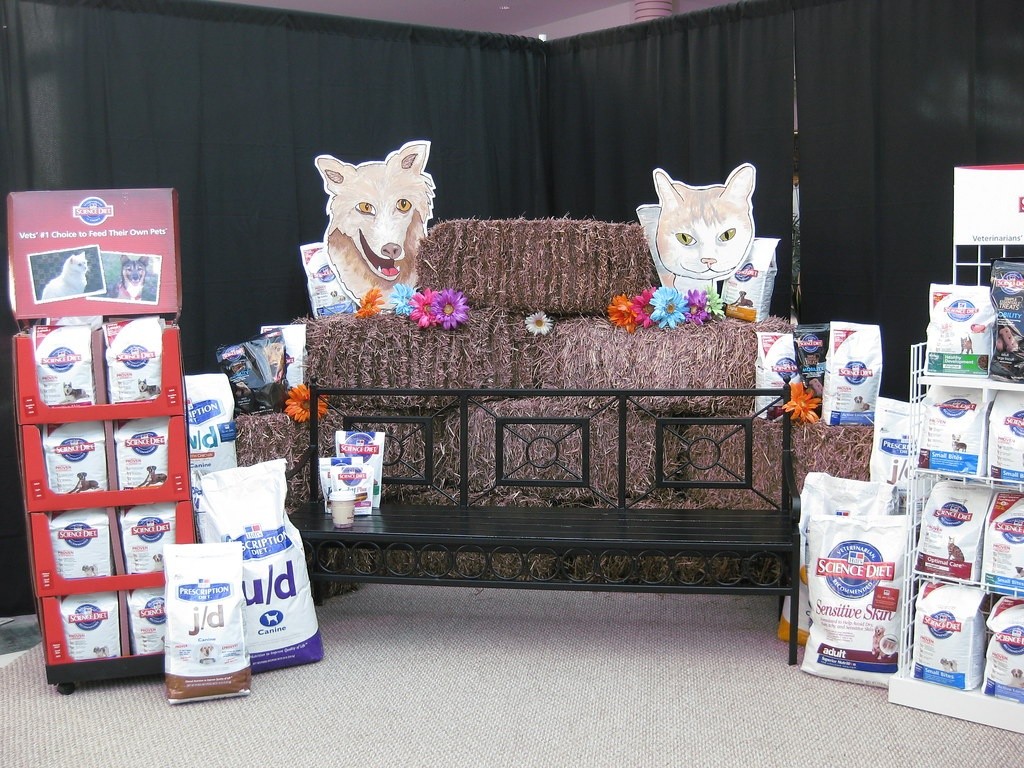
[329,490,355,529]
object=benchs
[282,374,804,666]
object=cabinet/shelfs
[887,161,1024,735]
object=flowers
[389,283,418,315]
[631,287,661,327]
[683,289,712,326]
[607,293,638,334]
[704,285,727,320]
[283,384,328,423]
[524,311,555,336]
[782,381,822,428]
[429,288,470,331]
[354,288,386,319]
[408,288,438,328]
[648,286,690,330]
